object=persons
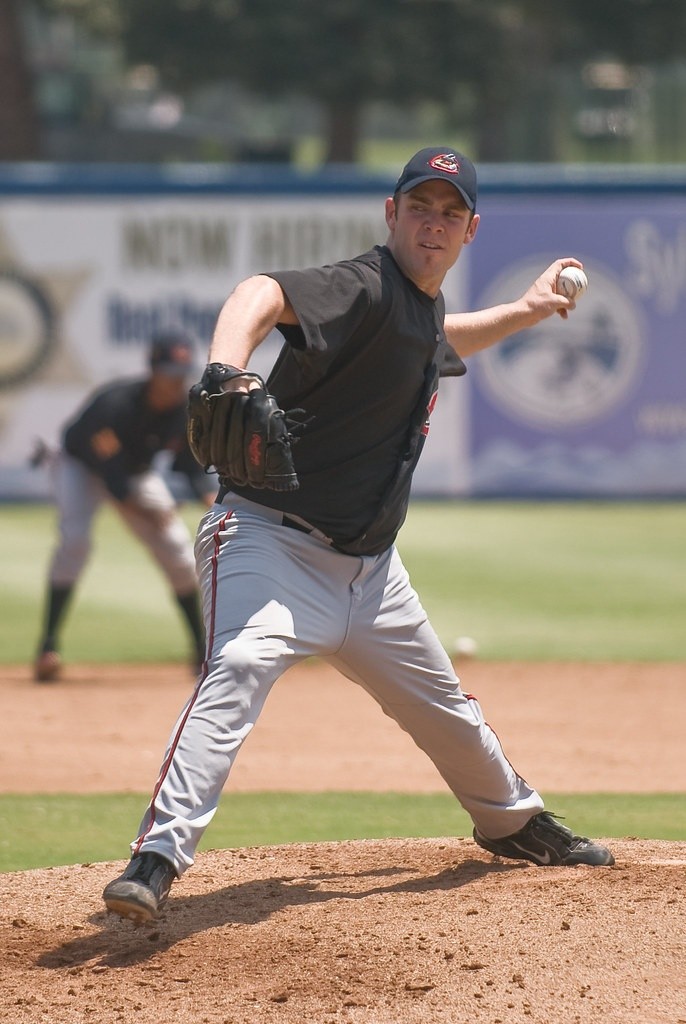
[33,323,218,678]
[101,146,616,922]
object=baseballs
[452,636,478,661]
[555,266,590,299]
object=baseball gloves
[186,361,302,492]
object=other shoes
[38,650,64,679]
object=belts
[214,486,341,550]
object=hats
[395,147,478,211]
[151,330,201,376]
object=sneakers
[103,852,177,924]
[473,811,615,866]
